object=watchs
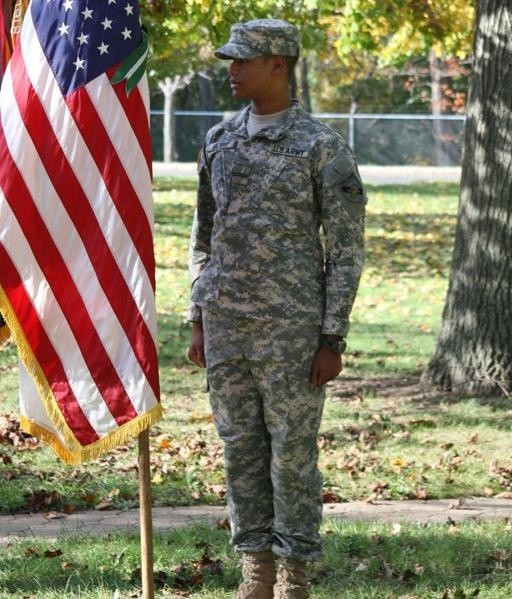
[323,335,347,355]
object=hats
[214,19,299,60]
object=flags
[1,0,165,468]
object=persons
[187,18,368,599]
